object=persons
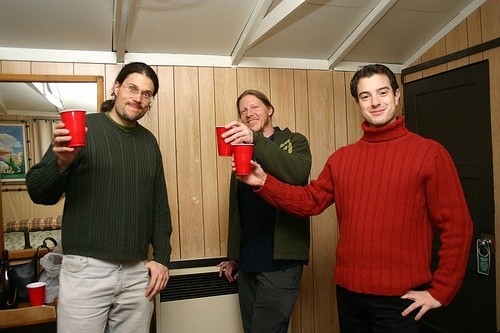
[215,89,313,333]
[232,64,473,333]
[24,62,174,333]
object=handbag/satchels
[8,236,62,303]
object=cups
[26,282,46,308]
[216,126,235,157]
[58,109,88,147]
[231,144,253,176]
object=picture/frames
[0,123,29,182]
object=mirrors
[0,73,104,260]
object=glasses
[119,83,154,103]
